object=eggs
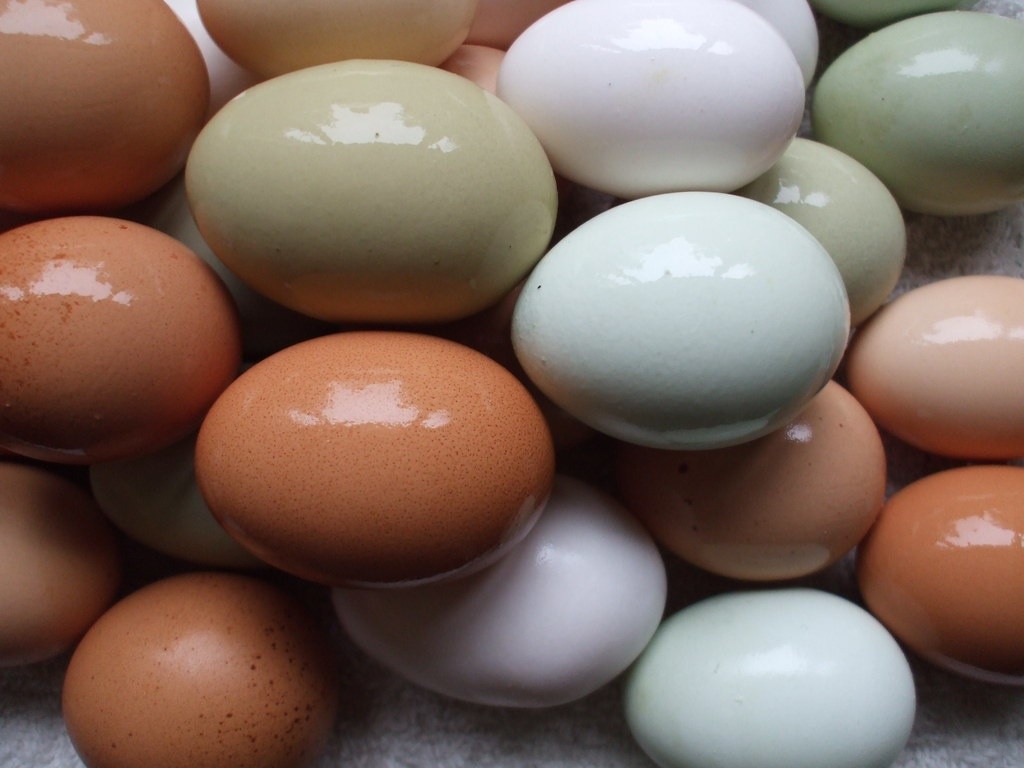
[0,0,1024,768]
